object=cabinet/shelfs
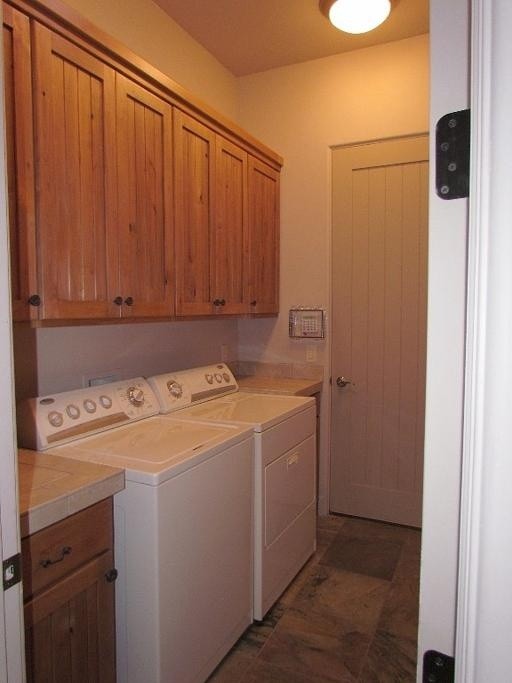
[20,494,118,681]
[247,153,281,315]
[172,105,252,323]
[31,19,176,329]
[1,0,39,327]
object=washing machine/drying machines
[146,360,317,622]
[17,376,254,683]
